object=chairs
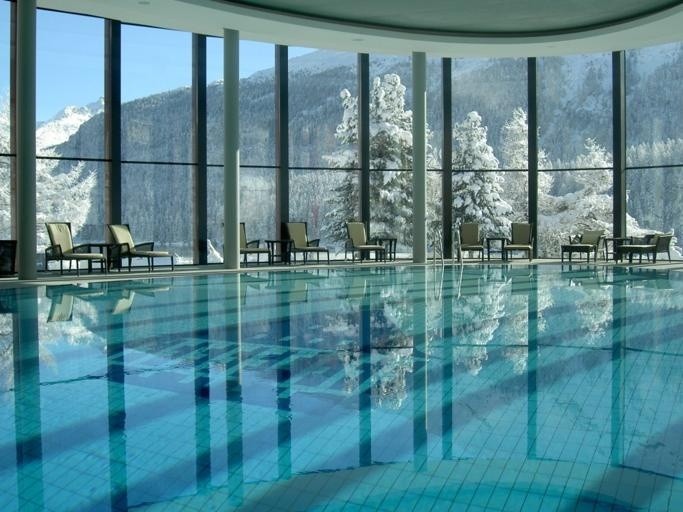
[44,221,108,277]
[454,223,484,262]
[280,270,329,307]
[510,264,536,295]
[105,280,170,316]
[223,272,267,305]
[561,265,608,289]
[629,267,674,291]
[46,282,108,324]
[107,224,174,273]
[337,267,385,303]
[222,222,272,267]
[505,223,535,262]
[618,234,673,263]
[561,230,605,262]
[454,265,487,295]
[344,221,386,263]
[281,221,330,264]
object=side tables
[373,238,397,262]
[379,266,398,286]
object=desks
[486,265,511,284]
[604,237,631,264]
[486,237,508,259]
[265,272,289,289]
[264,240,294,266]
[598,266,630,287]
